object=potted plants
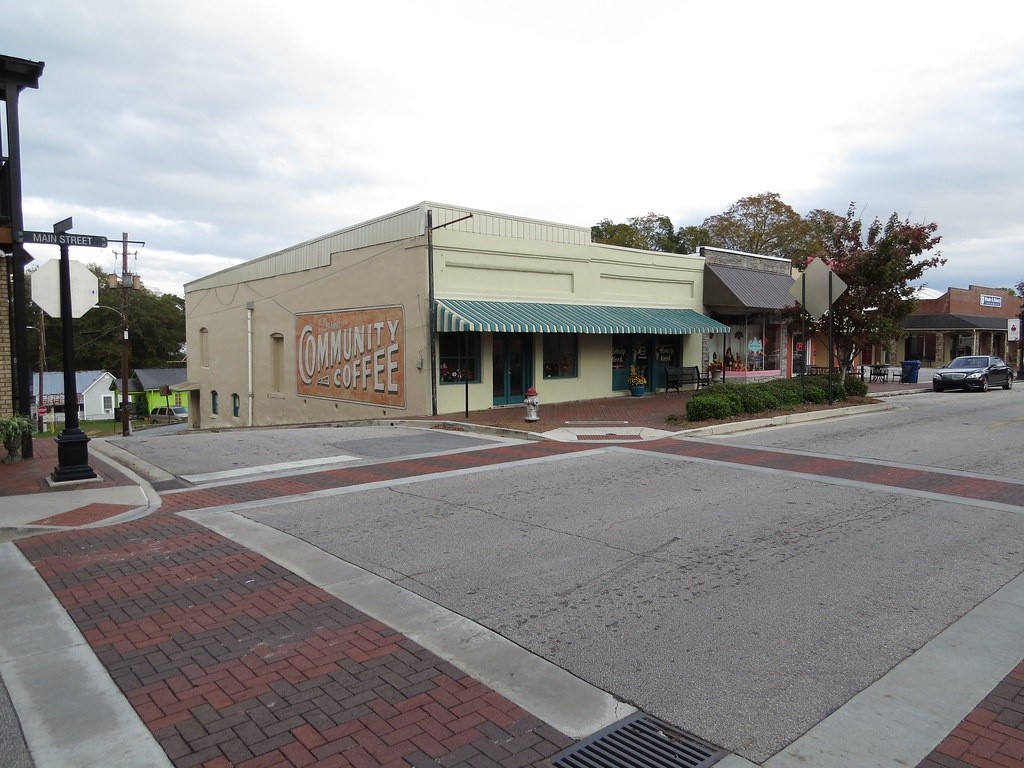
[0,411,37,464]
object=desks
[811,367,839,375]
[869,364,890,383]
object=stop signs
[37,405,48,416]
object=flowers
[707,360,723,374]
[627,368,647,386]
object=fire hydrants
[522,385,541,424]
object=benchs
[665,366,710,394]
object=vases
[712,369,721,383]
[631,384,645,397]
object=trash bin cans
[899,360,922,383]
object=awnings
[703,262,796,307]
[433,298,731,334]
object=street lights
[49,216,98,484]
[26,326,45,434]
[91,305,130,437]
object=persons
[724,347,734,367]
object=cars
[150,405,188,426]
[933,354,1014,393]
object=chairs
[806,365,867,380]
[892,366,913,385]
